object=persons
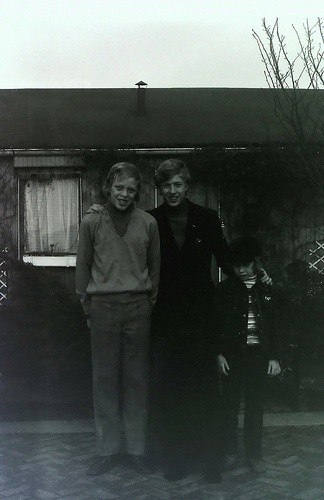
[209,238,295,473]
[73,167,160,475]
[88,157,274,479]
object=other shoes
[247,455,265,474]
[129,454,153,473]
[224,452,242,471]
[165,461,186,481]
[201,464,222,485]
[86,455,117,475]
